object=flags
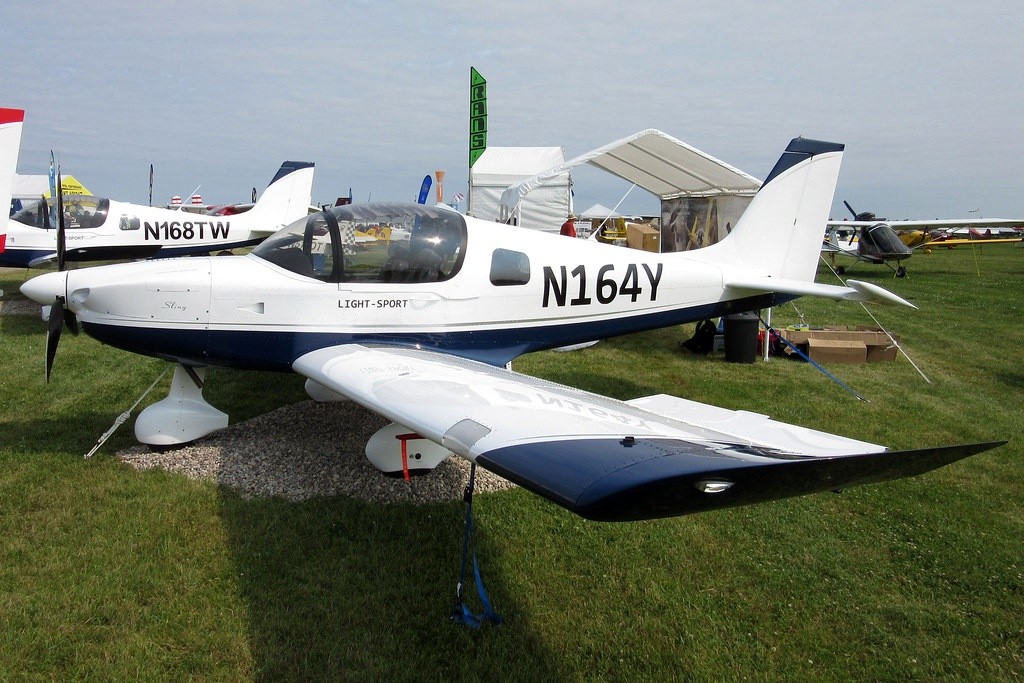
[172,196,182,204]
[191,195,202,204]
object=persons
[560,214,576,238]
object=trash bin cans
[720,313,760,363]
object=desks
[604,230,625,244]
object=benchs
[602,236,627,245]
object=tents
[498,128,775,253]
[466,145,571,231]
[21,173,92,199]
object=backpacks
[682,317,716,355]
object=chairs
[383,243,405,283]
[403,249,443,283]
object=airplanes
[17,138,1012,523]
[0,158,315,272]
[825,198,1024,279]
[849,232,1023,254]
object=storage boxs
[785,325,883,362]
[627,224,660,253]
[807,338,867,364]
[880,334,900,361]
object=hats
[565,213,577,220]
